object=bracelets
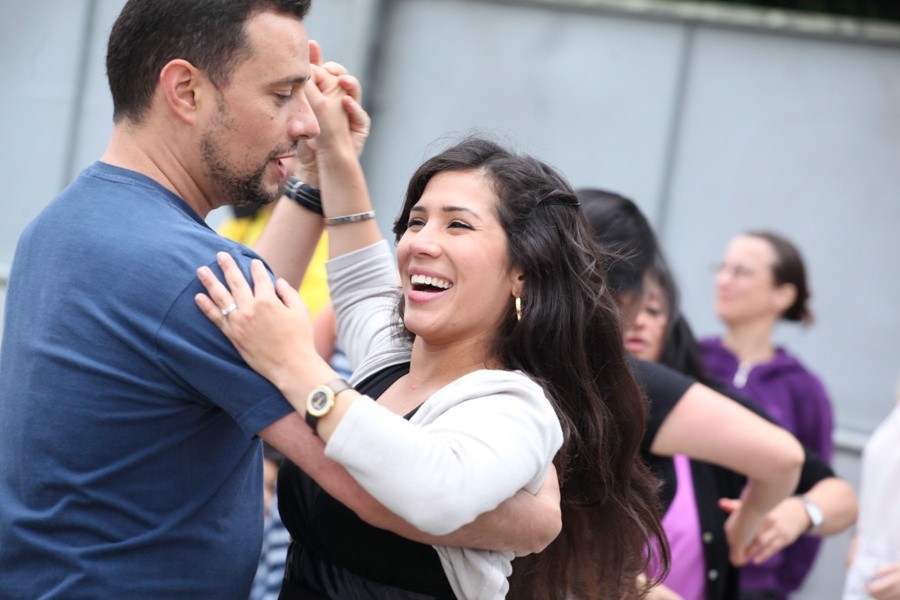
[323,210,375,225]
[283,176,323,216]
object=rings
[221,304,236,316]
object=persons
[574,189,857,600]
[0,0,563,600]
[194,64,672,600]
[843,381,900,600]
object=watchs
[798,494,821,540]
[306,379,351,437]
